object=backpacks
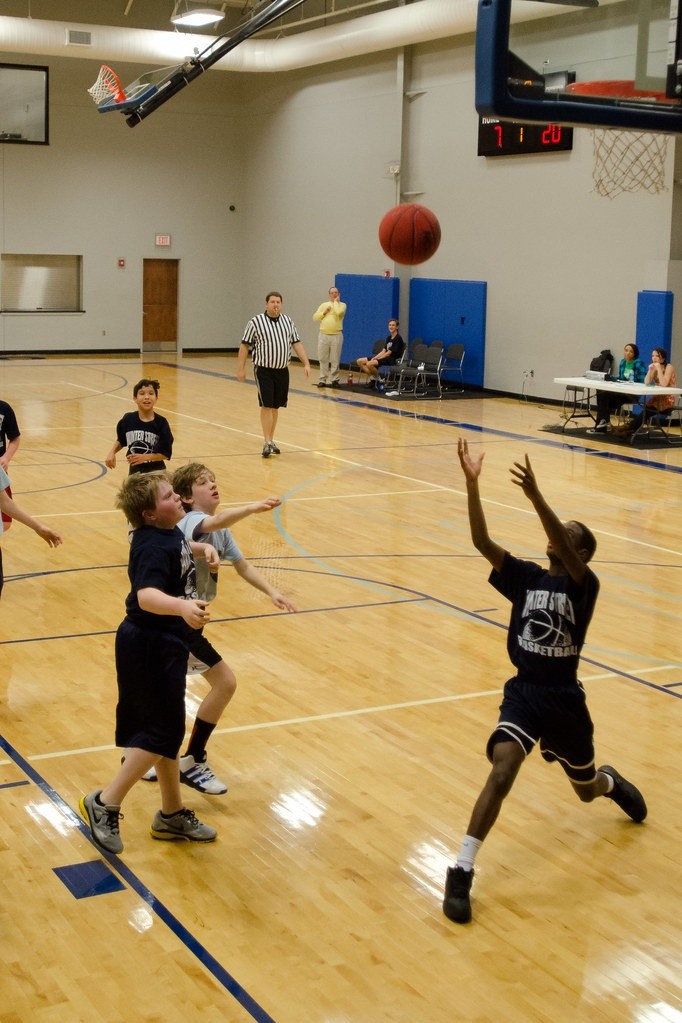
[591,350,615,373]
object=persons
[105,378,173,541]
[313,287,347,387]
[237,291,311,456]
[0,464,62,597]
[121,463,299,795]
[443,438,647,923]
[586,344,645,435]
[79,476,220,856]
[357,319,404,390]
[617,348,675,438]
[0,399,20,472]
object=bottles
[379,381,384,393]
[629,371,634,383]
[348,374,353,386]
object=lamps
[171,9,226,26]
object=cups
[418,363,425,371]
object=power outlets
[530,370,534,377]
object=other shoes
[318,382,326,387]
[332,382,342,388]
[612,424,634,437]
[262,440,280,456]
[364,380,383,391]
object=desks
[551,376,682,447]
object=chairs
[646,393,682,444]
[353,340,466,400]
[562,386,593,414]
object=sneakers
[180,751,227,795]
[121,755,158,781]
[444,863,476,925]
[597,418,611,428]
[78,790,124,854]
[586,428,607,434]
[599,764,648,823]
[149,806,217,843]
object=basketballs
[378,203,442,266]
